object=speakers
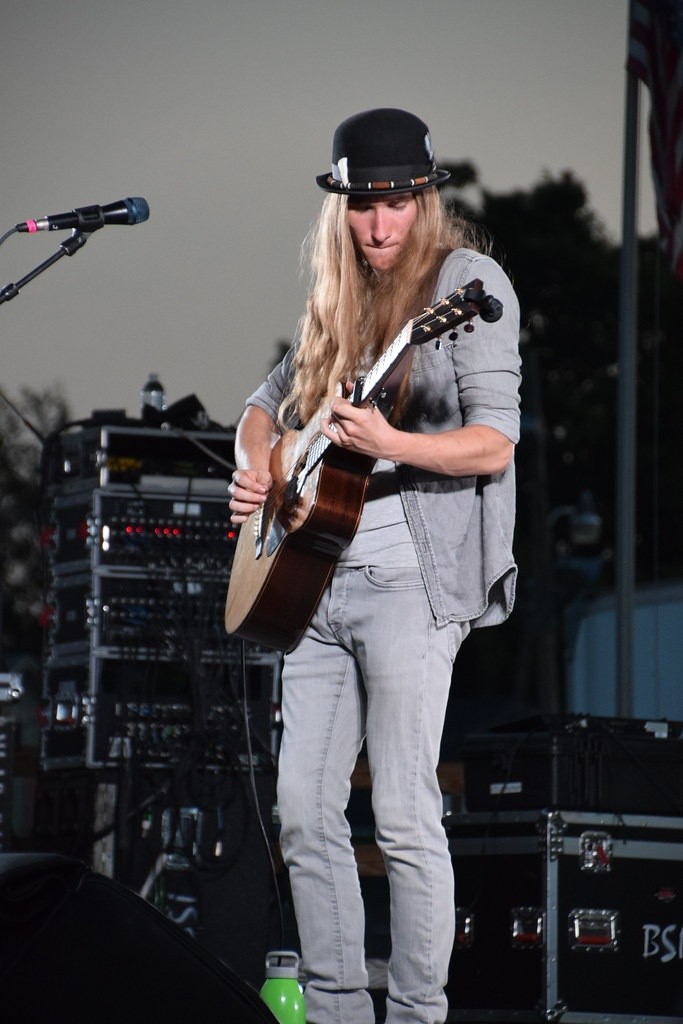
[0,852,280,1024]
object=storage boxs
[441,811,683,1024]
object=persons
[227,107,521,1024]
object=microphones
[15,196,150,233]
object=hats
[315,107,451,197]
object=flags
[626,0,683,277]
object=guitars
[221,277,505,656]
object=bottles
[259,951,307,1024]
[141,373,164,412]
